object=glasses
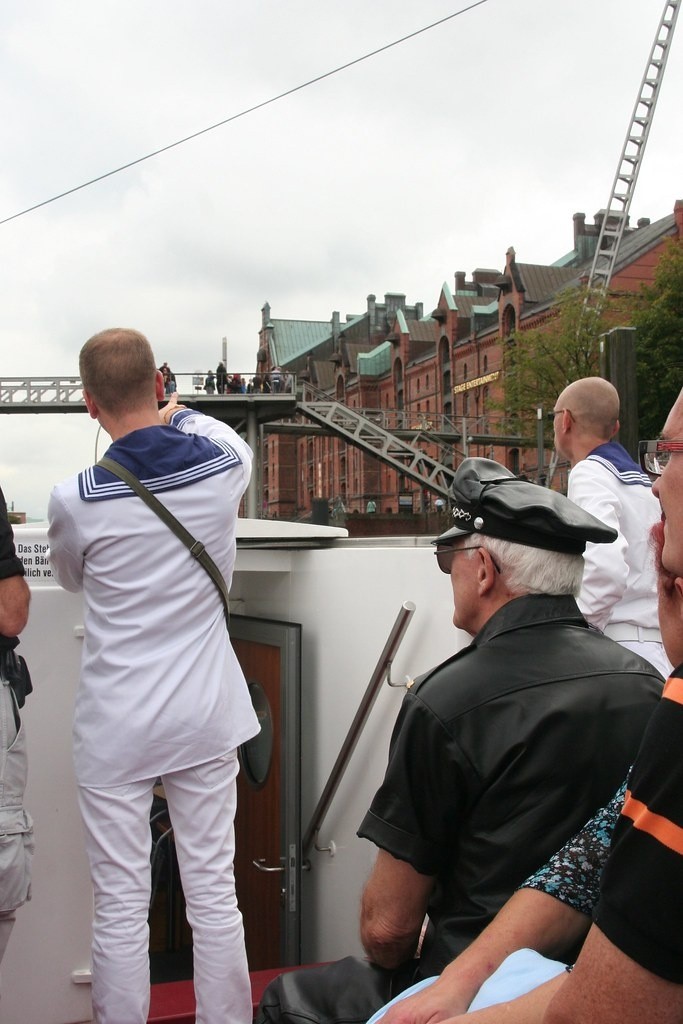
[434,544,501,574]
[548,409,576,423]
[639,440,683,477]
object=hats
[430,457,618,554]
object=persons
[542,380,683,1024]
[0,485,33,964]
[285,370,293,393]
[270,366,284,392]
[228,374,246,393]
[204,371,216,394]
[255,456,663,1024]
[216,361,228,393]
[246,373,271,393]
[373,519,683,1024]
[47,329,260,1024]
[158,361,176,394]
[647,452,661,473]
[553,377,677,684]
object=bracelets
[164,405,187,424]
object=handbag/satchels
[10,656,33,709]
